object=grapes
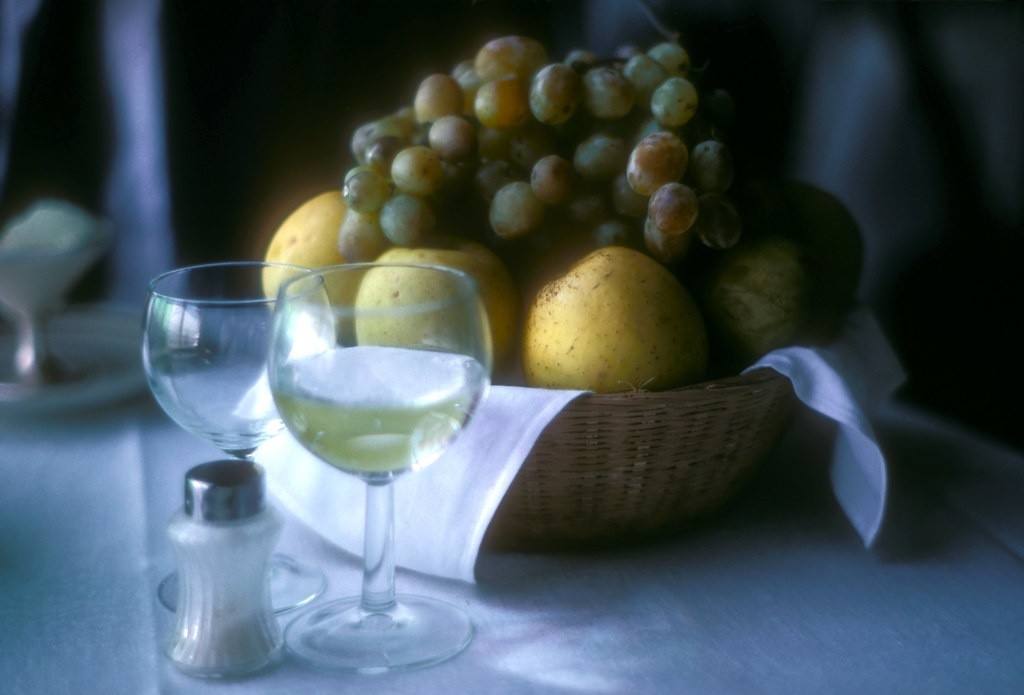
[336,36,747,255]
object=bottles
[164,461,282,682]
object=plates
[1,310,149,413]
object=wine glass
[264,259,494,679]
[141,260,338,625]
[0,218,117,382]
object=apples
[262,189,852,397]
